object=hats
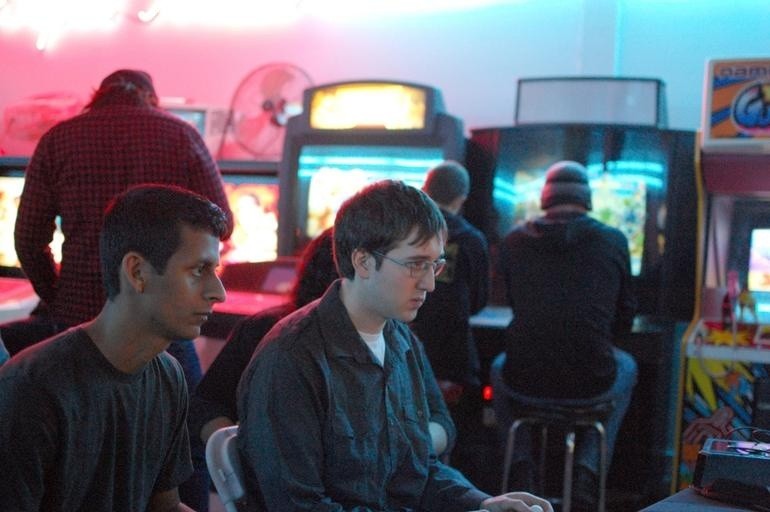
[540,160,593,211]
[98,68,160,107]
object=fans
[226,62,314,160]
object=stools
[501,403,618,511]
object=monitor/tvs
[209,171,278,266]
[0,164,65,279]
[746,227,770,291]
[290,141,445,256]
[479,144,671,280]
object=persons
[2,182,232,511]
[486,160,643,510]
[233,177,557,512]
[12,68,235,400]
[404,159,495,484]
[187,225,340,447]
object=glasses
[372,250,446,278]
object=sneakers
[507,459,600,508]
